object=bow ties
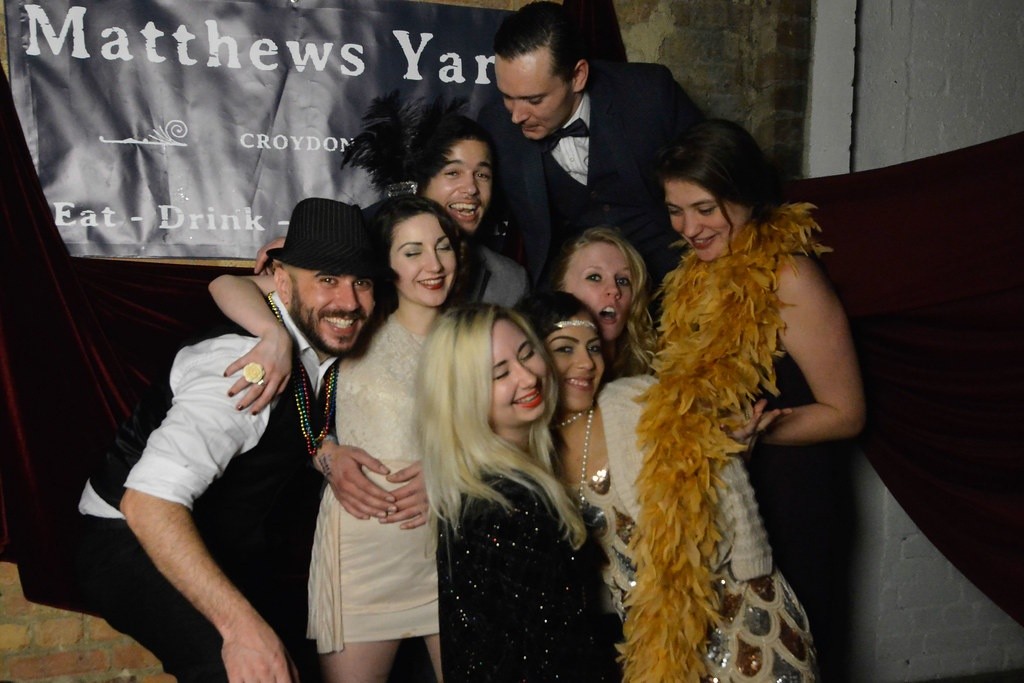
[536,118,589,154]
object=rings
[257,379,267,389]
[244,363,265,383]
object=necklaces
[267,291,340,460]
[551,400,594,522]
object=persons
[66,0,867,683]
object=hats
[266,197,399,280]
